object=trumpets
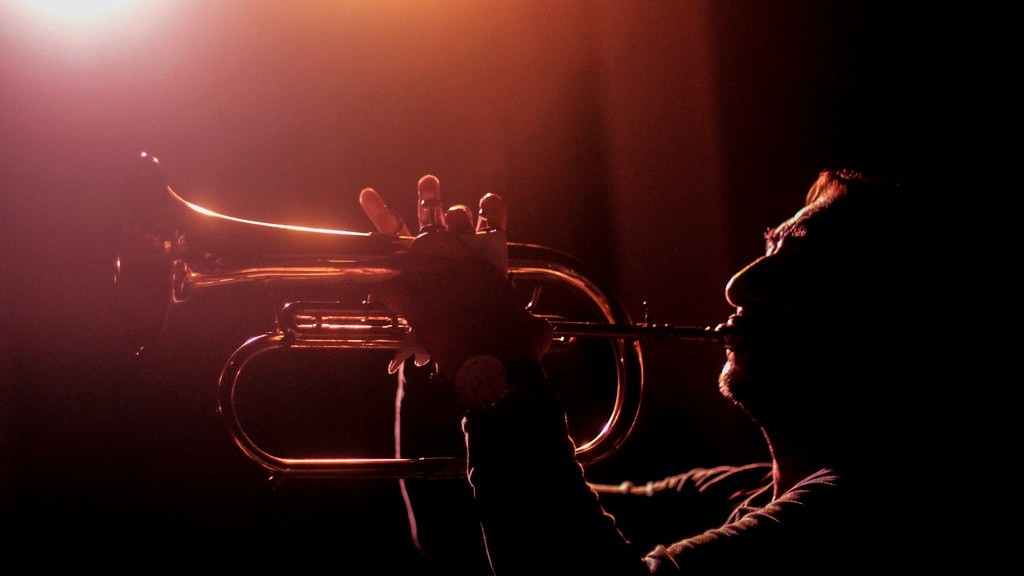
[113,148,733,474]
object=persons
[356,164,953,576]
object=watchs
[453,353,525,411]
